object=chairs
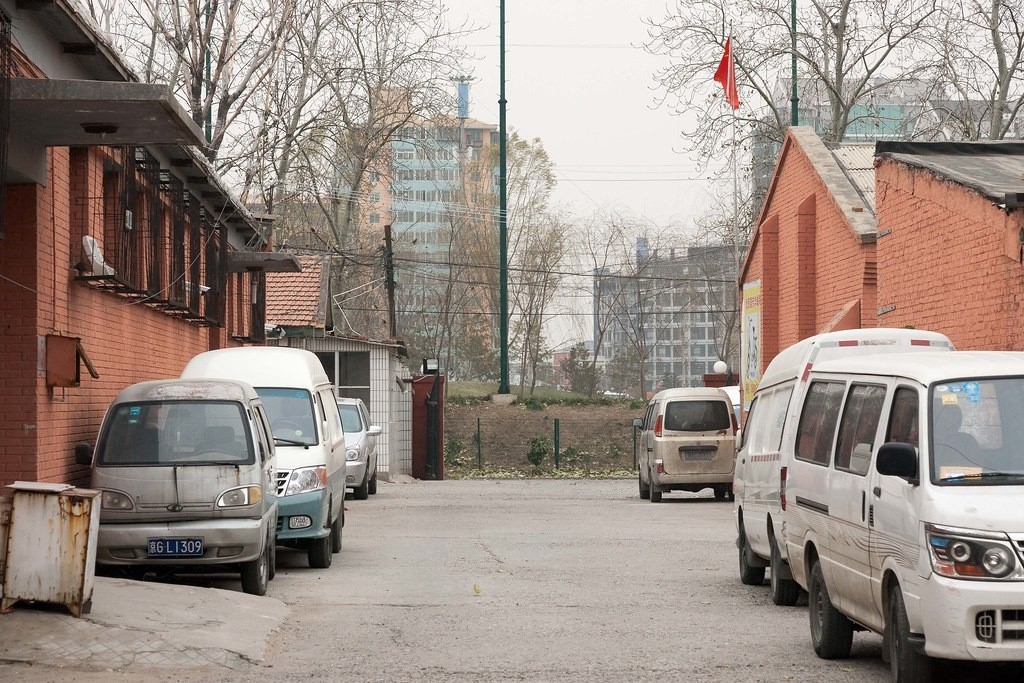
[194,425,245,458]
[914,398,985,467]
[133,428,171,461]
[270,395,313,438]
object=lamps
[420,357,440,375]
[714,360,728,373]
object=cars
[335,397,383,500]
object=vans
[785,350,1024,683]
[179,347,346,568]
[734,328,958,605]
[75,380,279,596]
[632,387,739,502]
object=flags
[714,38,739,108]
